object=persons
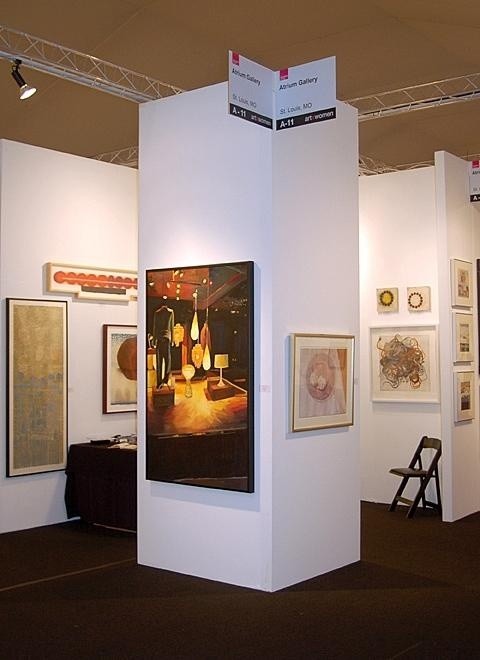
[153,305,175,389]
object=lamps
[9,59,37,100]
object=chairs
[388,435,442,518]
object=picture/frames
[146,261,253,494]
[450,258,473,308]
[6,297,69,477]
[102,323,137,413]
[454,370,475,422]
[290,332,356,431]
[369,324,440,404]
[453,311,472,363]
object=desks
[64,437,137,531]
